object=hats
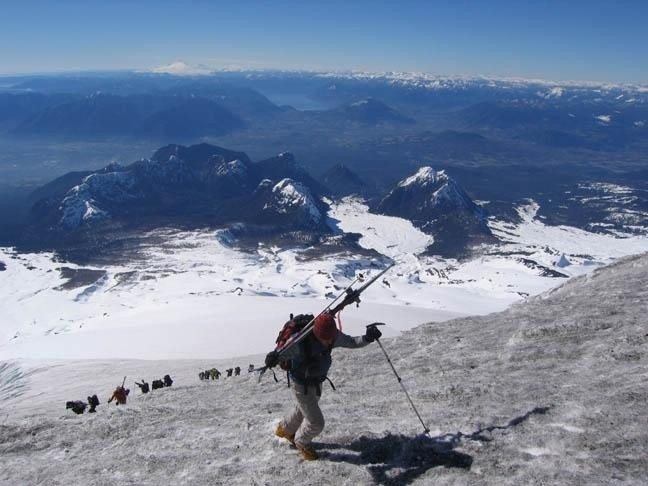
[314,314,337,340]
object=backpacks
[275,313,314,370]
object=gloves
[265,351,279,368]
[365,325,382,342]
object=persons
[198,364,255,380]
[65,374,173,415]
[266,313,382,461]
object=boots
[274,420,295,444]
[294,441,318,460]
